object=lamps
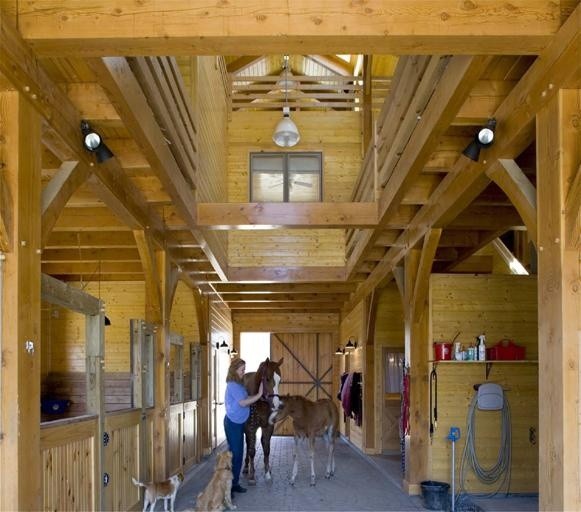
[216,335,238,355]
[461,118,498,164]
[273,52,300,147]
[335,334,357,356]
[79,120,114,164]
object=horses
[242,357,284,487]
[268,393,339,488]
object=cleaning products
[468,335,486,361]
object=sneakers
[231,485,247,493]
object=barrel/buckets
[433,343,453,360]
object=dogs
[132,472,184,512]
[183,451,238,512]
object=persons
[224,357,264,499]
